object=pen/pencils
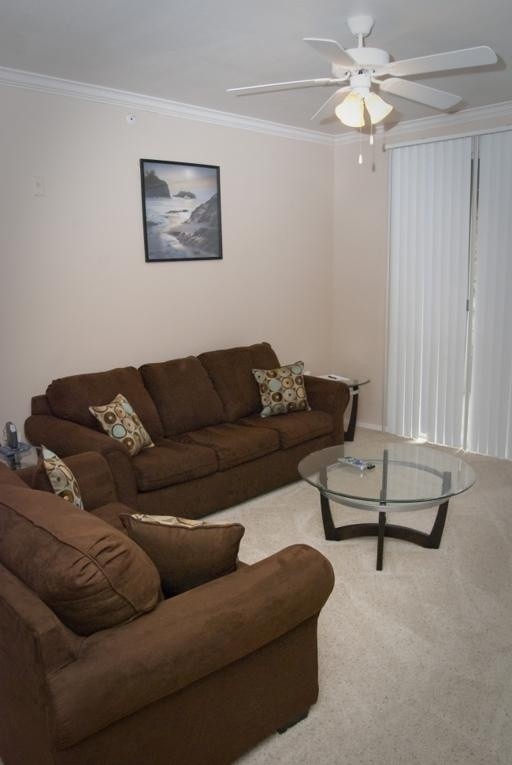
[328,376,337,379]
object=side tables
[304,371,371,442]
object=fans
[226,15,495,128]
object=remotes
[337,454,377,471]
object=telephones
[0,422,30,457]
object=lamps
[333,74,394,129]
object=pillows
[32,441,82,511]
[252,361,313,419]
[113,511,244,595]
[87,393,155,458]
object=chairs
[0,457,335,759]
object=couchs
[26,338,350,522]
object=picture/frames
[139,158,223,263]
